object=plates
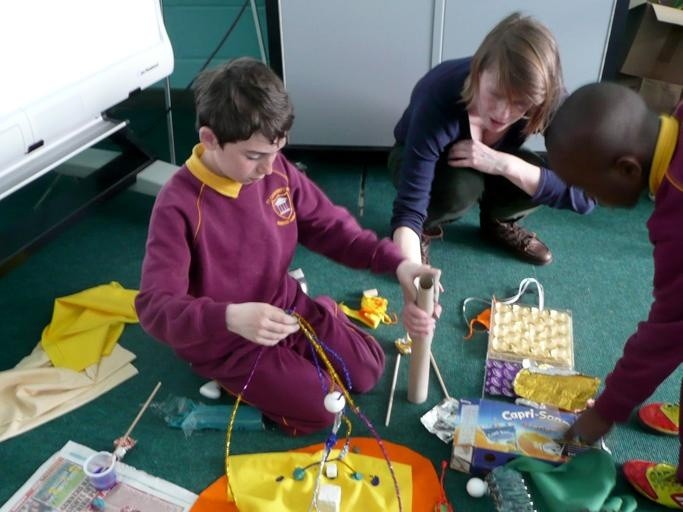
[274,0,616,146]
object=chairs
[621,460,683,510]
[639,402,680,434]
[480,221,552,265]
[422,228,444,265]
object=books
[600,1,683,116]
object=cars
[82,450,116,492]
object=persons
[136,60,445,434]
[557,76,683,507]
[384,10,599,337]
